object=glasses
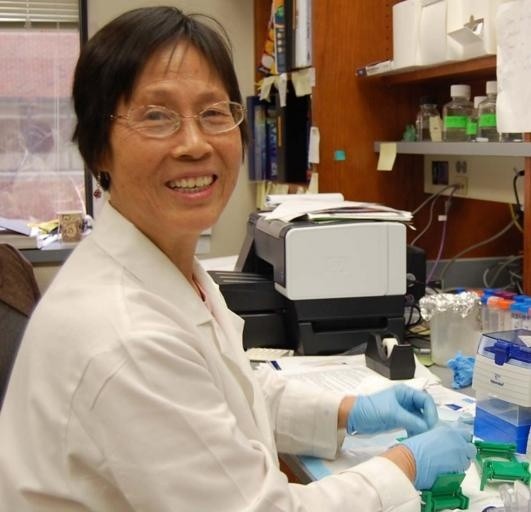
[108,99,245,140]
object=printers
[207,207,408,355]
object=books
[245,0,312,182]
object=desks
[251,336,477,484]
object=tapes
[382,337,399,359]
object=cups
[60,212,84,243]
[429,295,479,368]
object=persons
[1,5,477,512]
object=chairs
[0,242,42,415]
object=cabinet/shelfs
[253,0,413,210]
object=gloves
[346,384,439,435]
[388,426,477,490]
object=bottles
[457,287,530,332]
[402,81,524,142]
